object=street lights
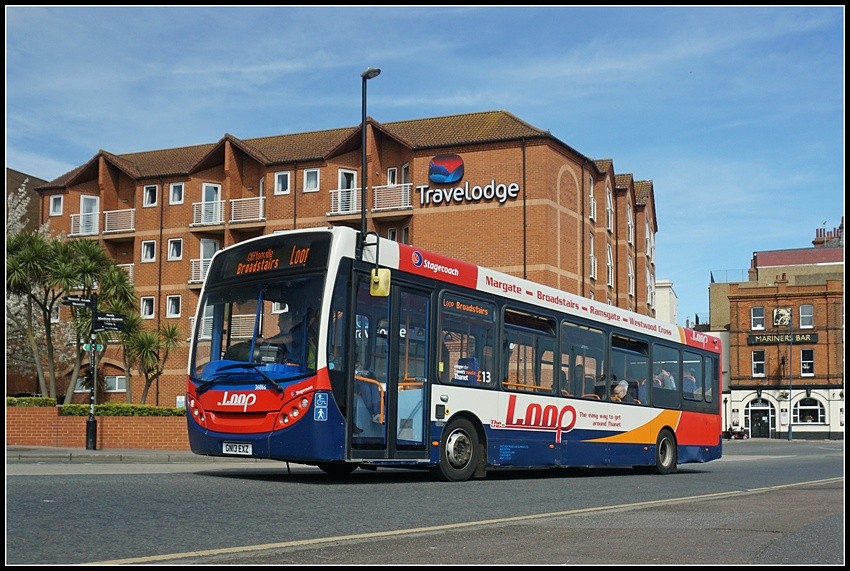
[357,67,382,240]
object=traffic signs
[60,295,93,308]
[84,344,103,352]
[93,320,124,331]
[96,312,128,321]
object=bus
[184,223,725,484]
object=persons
[256,306,385,433]
[802,412,816,422]
[550,364,696,403]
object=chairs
[457,358,479,384]
[546,374,698,404]
